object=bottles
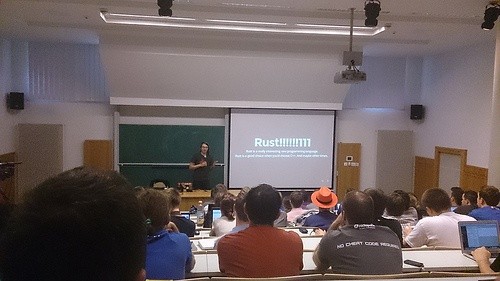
[197,201,204,226]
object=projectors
[334,69,367,84]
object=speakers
[9,92,24,110]
[410,105,423,120]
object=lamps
[363,2,381,27]
[156,0,173,17]
[480,6,500,31]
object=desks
[175,187,500,277]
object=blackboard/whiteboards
[113,115,229,189]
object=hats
[311,186,338,209]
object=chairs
[174,270,500,281]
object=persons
[189,141,218,190]
[0,166,499,281]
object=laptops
[458,220,500,263]
[198,239,214,249]
[181,213,190,220]
[211,207,222,222]
